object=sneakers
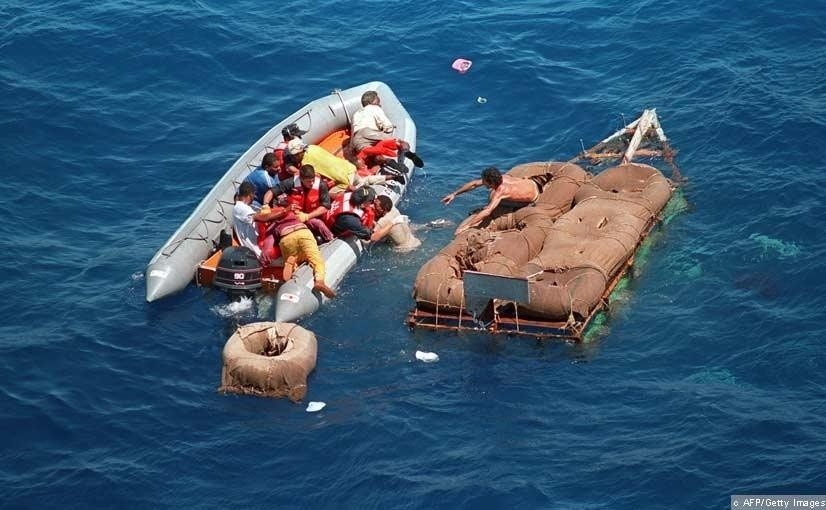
[405,150,424,168]
[385,158,410,173]
[313,280,337,300]
[281,256,297,282]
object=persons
[442,167,544,227]
[234,125,448,297]
[351,92,399,152]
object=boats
[395,104,690,345]
[135,75,422,330]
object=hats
[282,123,308,138]
